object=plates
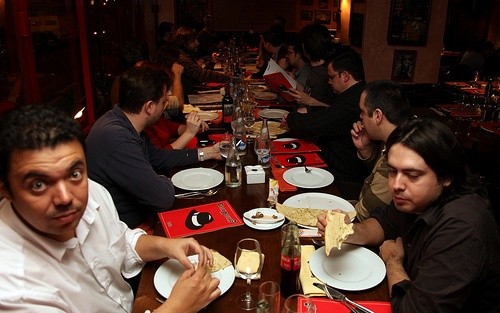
[282,166,335,188]
[282,192,357,229]
[243,208,285,231]
[171,167,224,190]
[309,243,387,291]
[246,80,289,135]
[185,112,218,121]
[153,253,235,298]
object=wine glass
[234,238,263,309]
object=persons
[0,106,221,313]
[315,115,500,313]
[86,23,411,239]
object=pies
[325,210,355,256]
[251,123,287,135]
[276,202,357,226]
[182,104,202,113]
[206,248,232,273]
[255,92,275,98]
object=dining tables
[134,38,391,313]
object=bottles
[220,35,257,157]
[224,136,242,188]
[257,118,270,169]
[278,221,302,297]
[484,79,493,100]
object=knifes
[313,283,374,313]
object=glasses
[287,50,296,56]
[327,71,344,80]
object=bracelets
[198,147,205,162]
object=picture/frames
[386,0,433,46]
[300,0,338,23]
[391,49,417,82]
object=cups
[258,281,281,313]
[282,294,314,313]
